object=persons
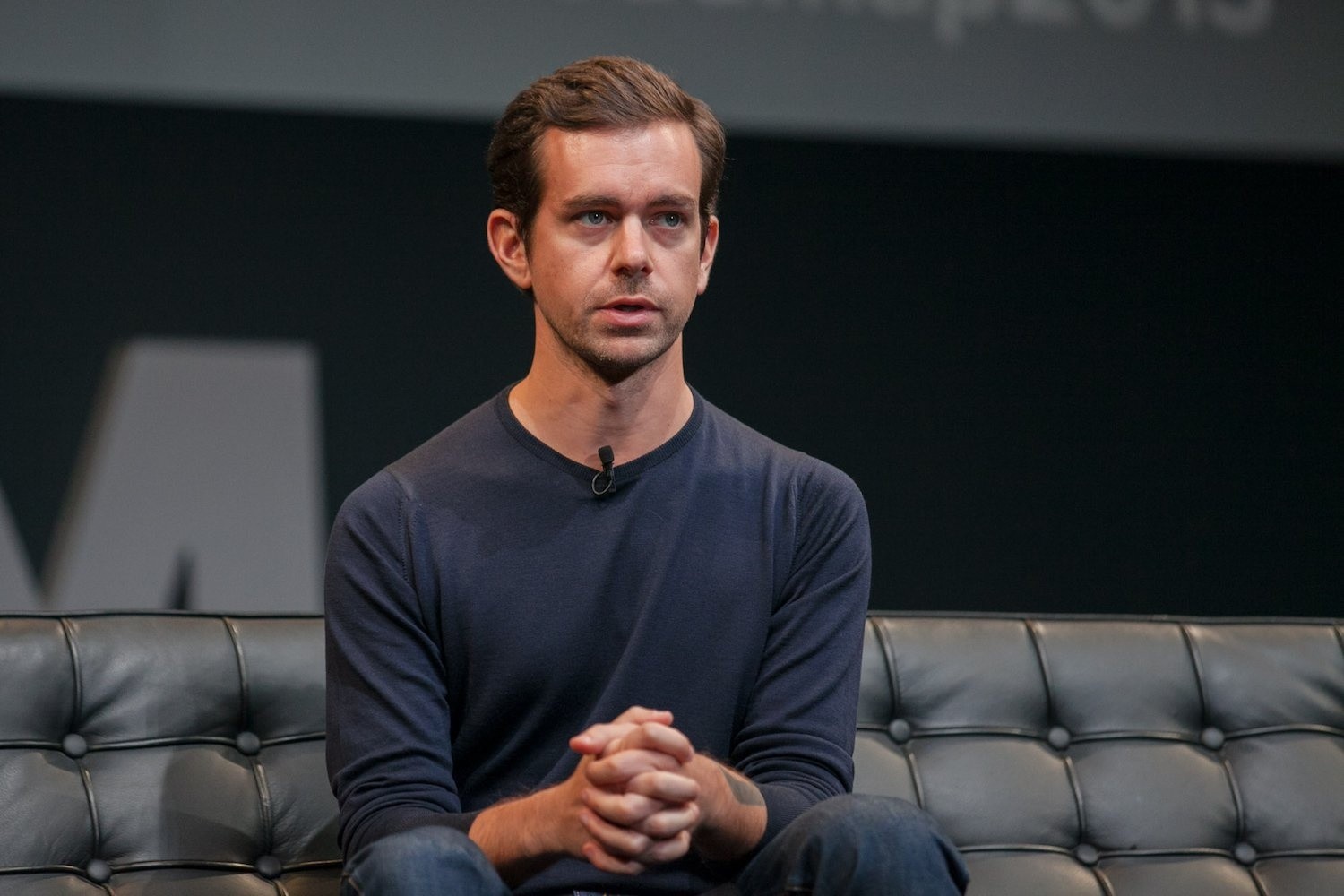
[315,49,980,896]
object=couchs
[0,599,1343,895]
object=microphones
[592,445,616,498]
